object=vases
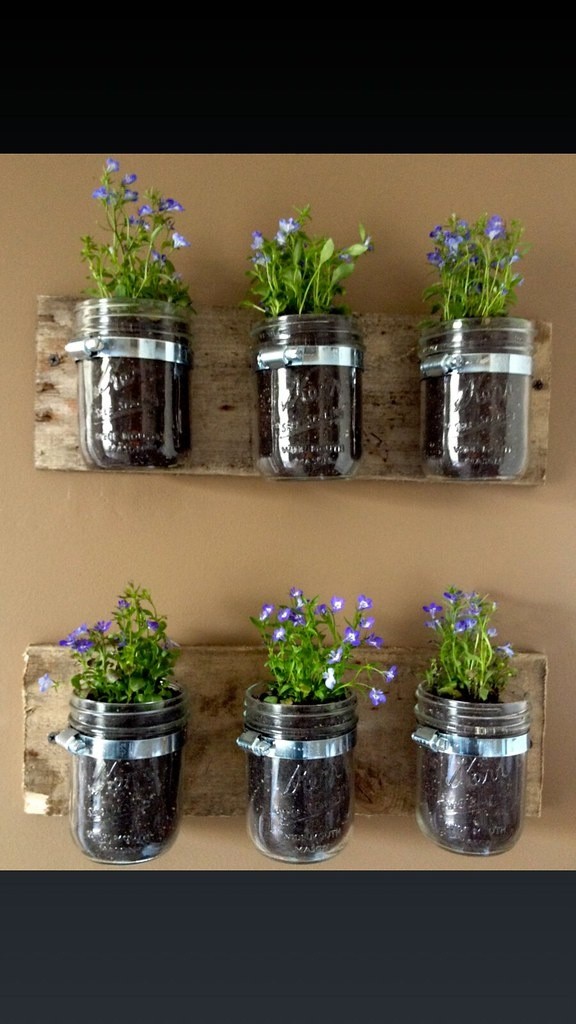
[73,298,193,468]
[242,682,358,865]
[68,677,188,865]
[250,315,369,483]
[413,681,531,855]
[416,316,533,480]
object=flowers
[38,582,182,709]
[249,586,396,706]
[77,157,198,323]
[421,582,519,701]
[235,202,377,318]
[414,213,533,332]
